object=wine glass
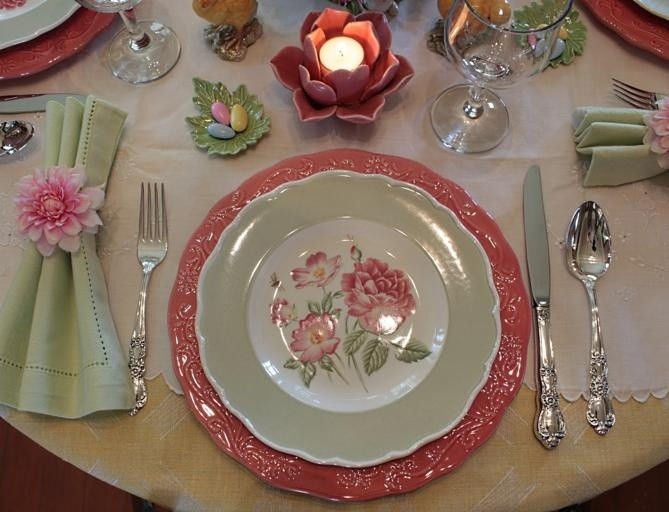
[74,0,183,87]
[424,1,574,157]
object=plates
[0,6,118,87]
[0,1,83,54]
[161,149,534,506]
[582,0,669,63]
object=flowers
[269,251,431,393]
[13,163,105,257]
[642,100,668,169]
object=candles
[317,35,365,80]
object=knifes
[1,90,87,115]
[520,161,569,454]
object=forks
[122,180,171,419]
[610,75,669,111]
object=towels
[570,106,669,189]
[1,94,135,421]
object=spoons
[562,200,618,439]
[0,117,36,158]
